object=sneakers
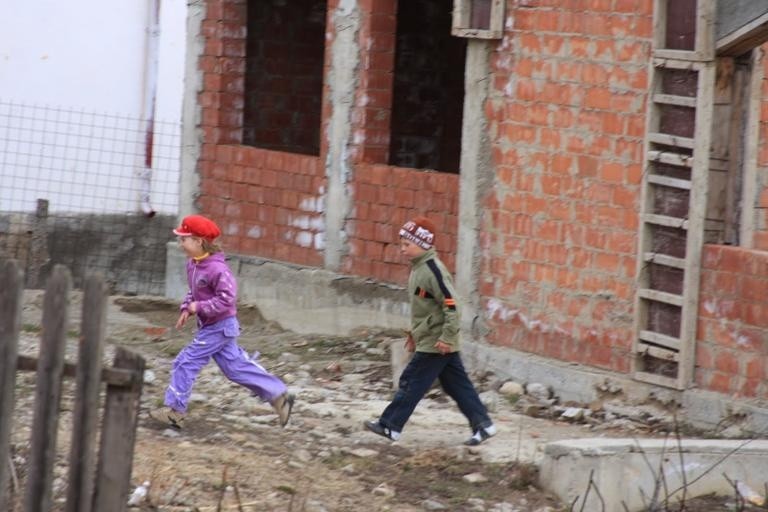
[150,408,186,428]
[364,419,402,441]
[465,424,496,444]
[271,393,295,426]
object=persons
[148,214,297,431]
[363,215,500,448]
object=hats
[398,216,435,250]
[173,215,221,240]
